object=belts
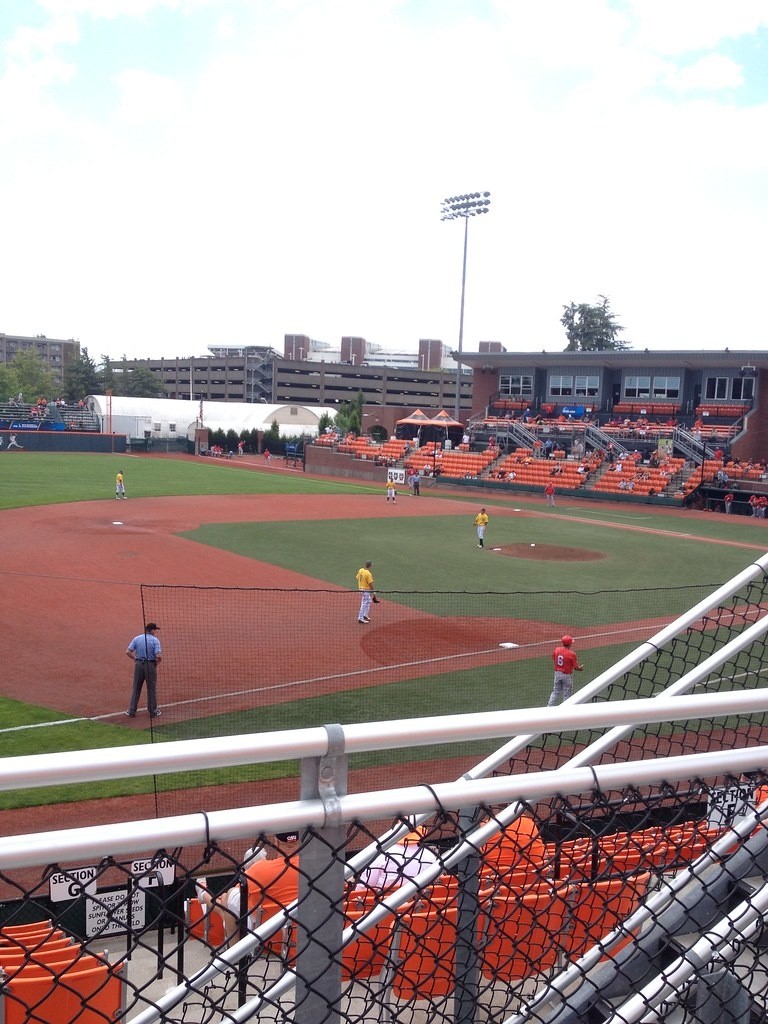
[136,659,156,662]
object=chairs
[315,397,764,500]
[1,815,768,1024]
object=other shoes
[122,497,127,500]
[393,501,397,504]
[477,545,484,547]
[387,501,389,503]
[116,497,120,500]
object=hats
[562,635,575,645]
[144,623,160,631]
[277,832,299,843]
[364,560,374,569]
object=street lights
[439,191,493,423]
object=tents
[396,409,464,439]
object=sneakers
[195,877,207,905]
[359,620,369,624]
[124,711,135,717]
[150,710,162,718]
[363,618,370,621]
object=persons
[548,635,583,706]
[196,830,298,963]
[70,420,74,430]
[465,471,472,479]
[200,440,245,459]
[415,447,443,458]
[115,470,128,500]
[125,623,162,718]
[480,803,548,871]
[386,478,397,503]
[79,399,84,410]
[407,463,442,496]
[9,395,65,418]
[325,425,410,467]
[462,389,768,518]
[356,561,376,624]
[354,816,440,891]
[264,449,271,466]
[474,508,488,547]
[545,482,555,507]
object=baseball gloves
[372,596,380,603]
